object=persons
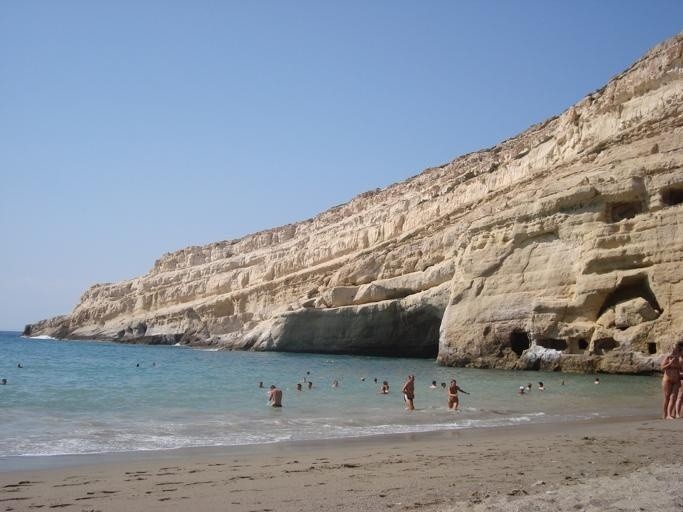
[660,341,683,420]
[558,380,567,386]
[516,382,545,395]
[594,378,601,384]
[257,371,471,412]
